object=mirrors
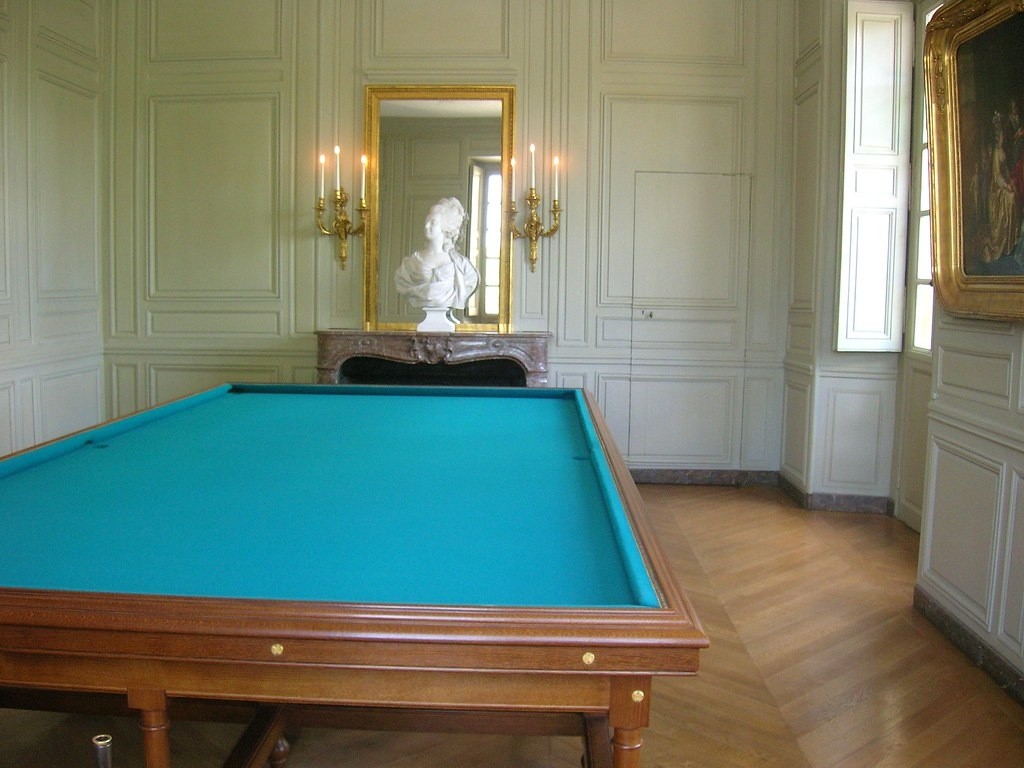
[365,84,517,334]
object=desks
[0,381,710,768]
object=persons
[394,197,478,311]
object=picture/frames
[924,1,1024,323]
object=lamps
[316,146,369,270]
[508,144,560,274]
